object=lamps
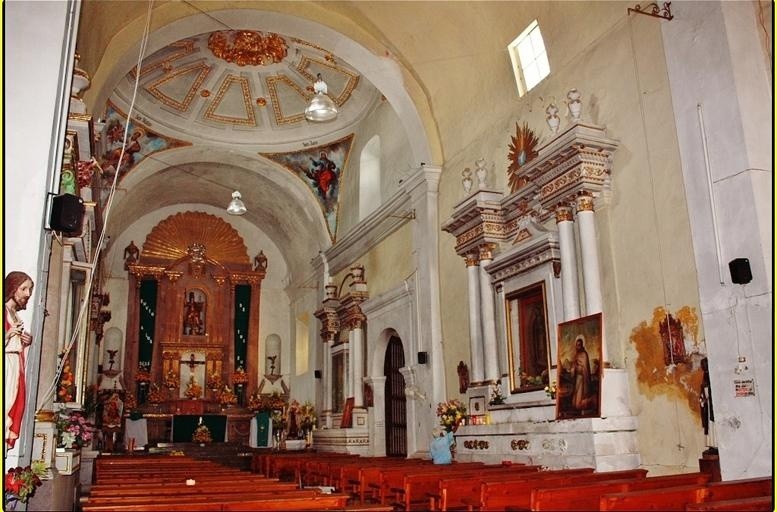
[304,72,338,122]
[227,189,247,216]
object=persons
[431,417,462,464]
[564,333,593,411]
[698,358,717,453]
[530,303,547,375]
[184,291,200,329]
[306,148,337,201]
[111,126,145,166]
[3,272,35,457]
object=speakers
[728,257,753,285]
[51,193,85,238]
[418,352,427,364]
[315,370,322,378]
[98,365,102,373]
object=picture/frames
[555,312,602,420]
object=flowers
[58,344,74,403]
[60,412,94,450]
[437,398,467,430]
[5,465,41,503]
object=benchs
[82,451,775,511]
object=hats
[432,427,442,438]
